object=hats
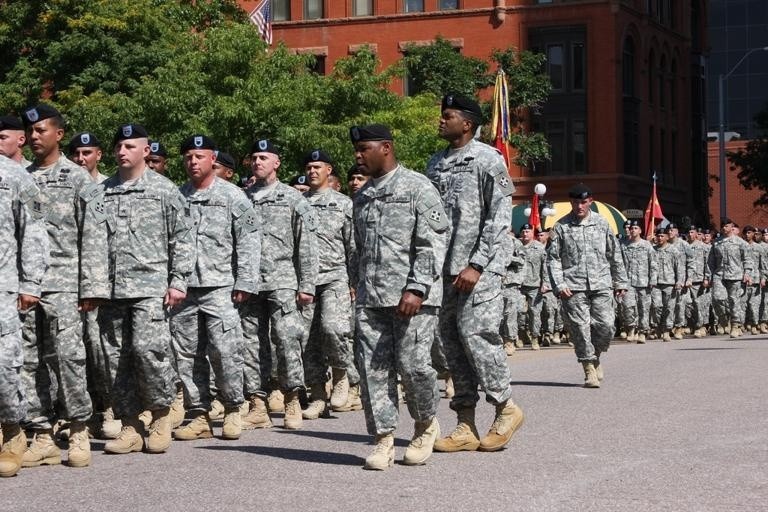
[250,139,279,155]
[0,115,25,132]
[569,185,591,200]
[347,165,362,182]
[23,103,58,127]
[69,132,100,154]
[148,142,166,158]
[113,125,148,149]
[656,223,677,235]
[441,90,483,117]
[623,220,642,229]
[517,223,549,239]
[290,175,310,186]
[350,125,393,143]
[213,150,235,170]
[688,219,768,239]
[304,149,330,166]
[180,134,216,154]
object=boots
[0,409,174,477]
[404,416,441,466]
[172,370,362,440]
[503,330,574,355]
[365,433,395,471]
[582,357,603,387]
[620,321,768,343]
[481,398,524,451]
[445,376,454,397]
[434,409,481,451]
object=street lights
[717,44,768,230]
[523,182,557,232]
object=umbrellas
[490,69,512,169]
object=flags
[249,0,272,53]
